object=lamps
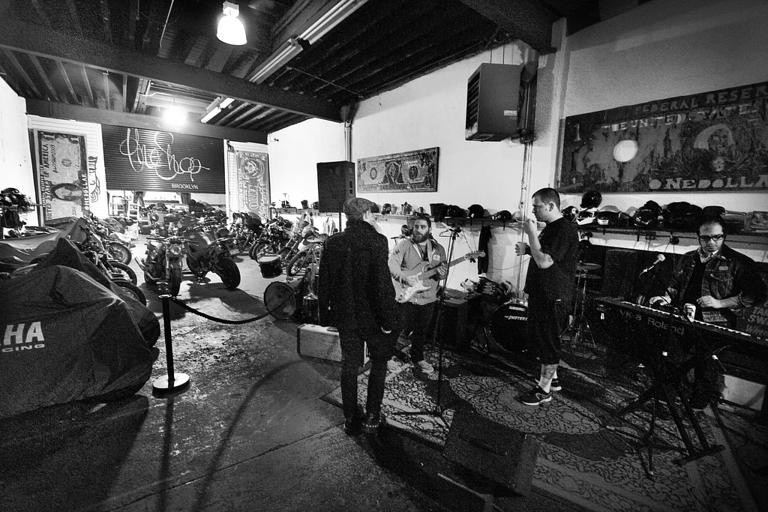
[217,1,247,45]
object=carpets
[321,345,759,512]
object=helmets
[467,205,485,218]
[598,206,622,227]
[633,206,657,229]
[576,207,598,225]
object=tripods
[392,239,458,430]
[626,315,687,476]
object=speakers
[316,161,355,212]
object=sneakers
[520,387,552,405]
[535,374,562,391]
[366,407,380,426]
[344,404,363,433]
[416,360,434,373]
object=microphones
[450,227,461,239]
[640,254,666,276]
[673,309,686,314]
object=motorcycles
[0,187,134,288]
[173,220,244,290]
[137,199,338,277]
[0,230,146,310]
[134,234,203,293]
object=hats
[344,198,374,215]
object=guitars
[393,251,485,304]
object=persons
[516,187,579,405]
[317,198,396,435]
[388,215,448,373]
[643,216,768,410]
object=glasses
[697,234,726,241]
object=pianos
[594,297,767,386]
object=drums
[488,299,529,352]
[265,278,310,320]
[474,277,507,300]
[258,256,282,278]
